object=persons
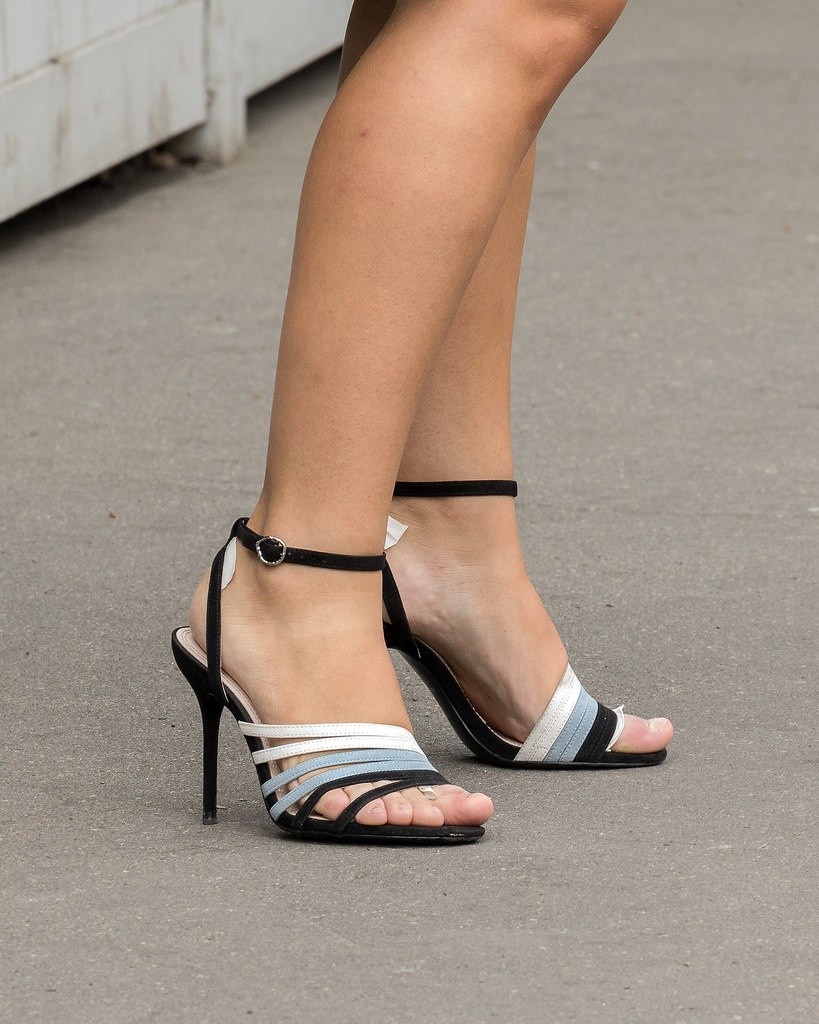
[170,1,673,849]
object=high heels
[380,479,668,770]
[171,515,485,845]
